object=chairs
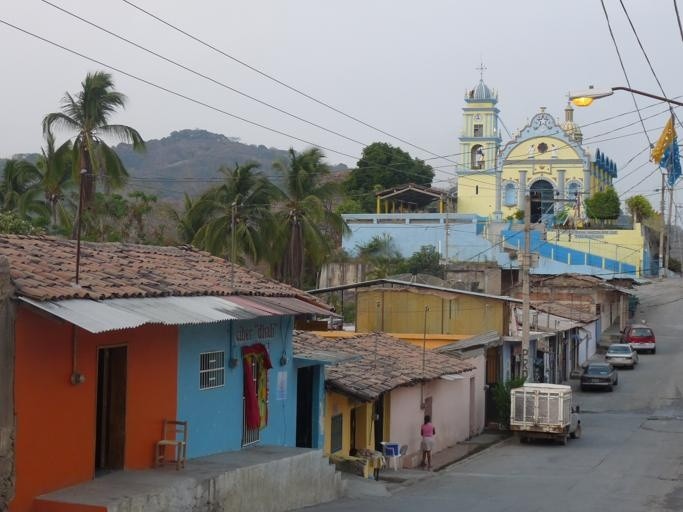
[151,417,191,469]
[380,441,402,472]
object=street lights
[568,85,683,108]
[653,188,672,275]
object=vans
[620,325,656,353]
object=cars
[605,343,638,368]
[579,362,618,391]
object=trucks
[508,381,582,445]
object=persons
[421,415,436,471]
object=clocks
[474,113,482,123]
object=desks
[356,449,382,482]
